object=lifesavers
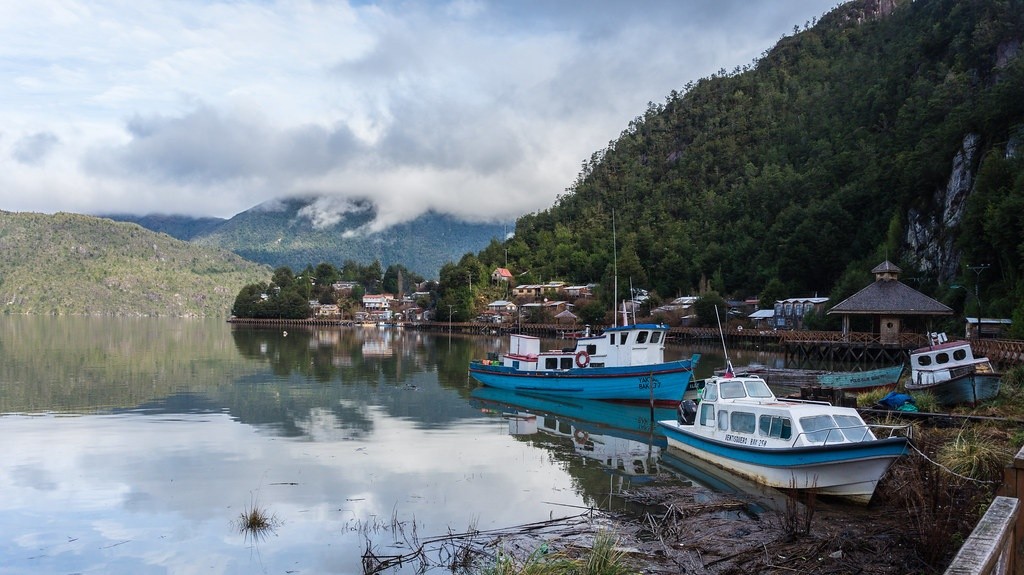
[574,429,588,444]
[576,351,589,368]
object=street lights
[950,286,982,340]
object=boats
[904,330,1004,409]
[470,386,679,488]
[470,208,701,408]
[716,364,906,391]
[657,304,911,505]
